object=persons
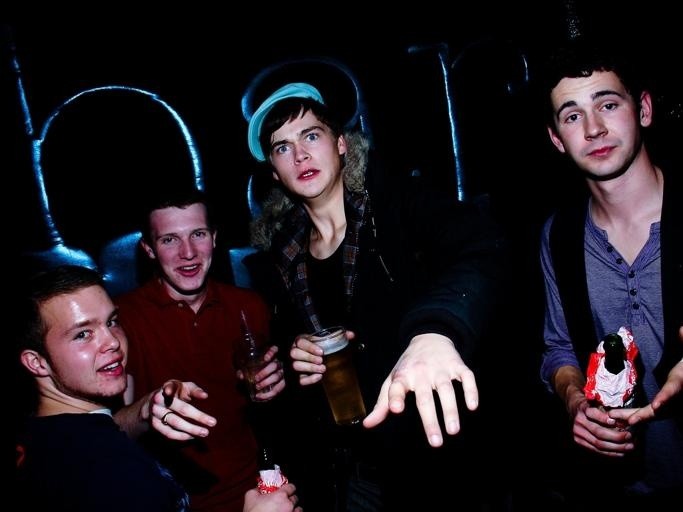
[0,255,304,512]
[97,182,302,511]
[509,34,682,510]
[244,79,528,509]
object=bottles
[236,309,275,403]
[594,334,638,458]
[254,446,287,495]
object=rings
[158,409,174,424]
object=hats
[247,80,324,163]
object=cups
[306,326,367,427]
